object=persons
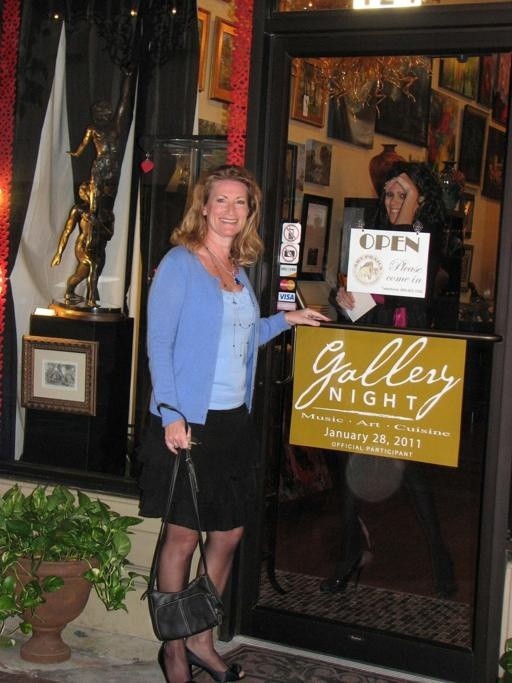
[317,159,465,597]
[136,161,335,683]
[62,59,141,310]
[50,178,94,306]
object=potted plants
[0,481,152,664]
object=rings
[339,299,344,304]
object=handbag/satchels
[147,574,225,642]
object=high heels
[157,641,196,683]
[429,551,457,596]
[183,644,246,683]
[320,549,371,593]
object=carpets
[189,643,422,682]
[258,567,473,652]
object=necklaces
[202,241,241,287]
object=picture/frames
[21,334,100,416]
